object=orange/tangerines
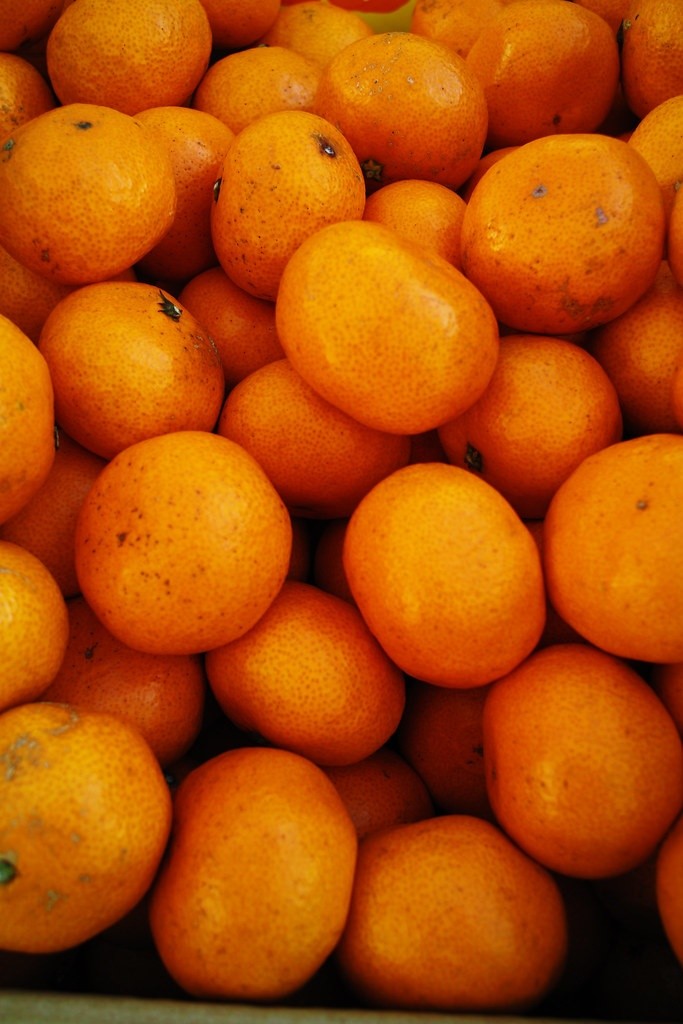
[0,0,683,1014]
